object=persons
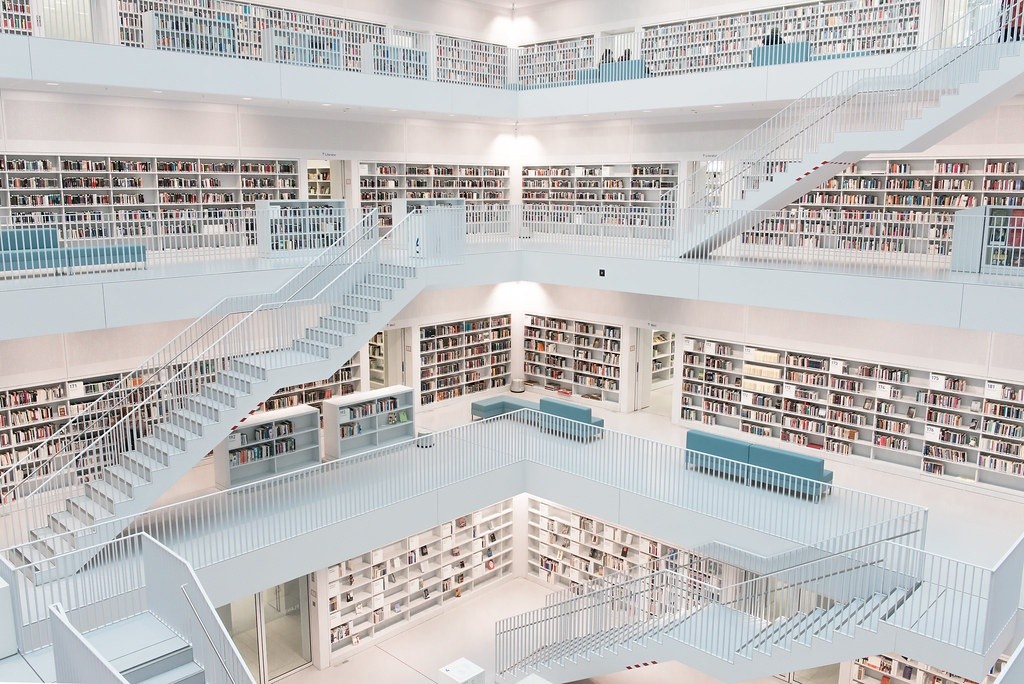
[618,49,630,61]
[762,27,786,45]
[601,49,615,62]
[998,0,1024,42]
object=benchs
[0,228,147,279]
[600,59,646,81]
[685,430,834,503]
[752,41,811,65]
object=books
[679,338,1024,480]
[854,655,994,684]
[641,11,782,71]
[329,512,496,646]
[653,333,675,372]
[743,161,1024,268]
[420,316,511,406]
[522,167,675,226]
[1,0,32,36]
[784,0,919,53]
[523,316,620,401]
[360,165,508,222]
[437,38,507,89]
[540,516,720,615]
[229,333,408,466]
[0,158,344,251]
[0,364,216,504]
[519,38,594,84]
[119,0,427,80]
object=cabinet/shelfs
[307,497,513,669]
[0,152,300,239]
[520,161,680,229]
[0,342,360,516]
[472,395,604,441]
[735,155,1024,253]
[392,197,466,264]
[850,651,1007,684]
[405,313,511,413]
[322,384,416,463]
[255,199,347,251]
[369,330,388,389]
[524,497,725,617]
[213,404,321,494]
[358,160,511,231]
[0,0,921,88]
[670,335,1024,504]
[651,330,675,390]
[951,203,1023,276]
[524,312,623,410]
[307,168,332,200]
